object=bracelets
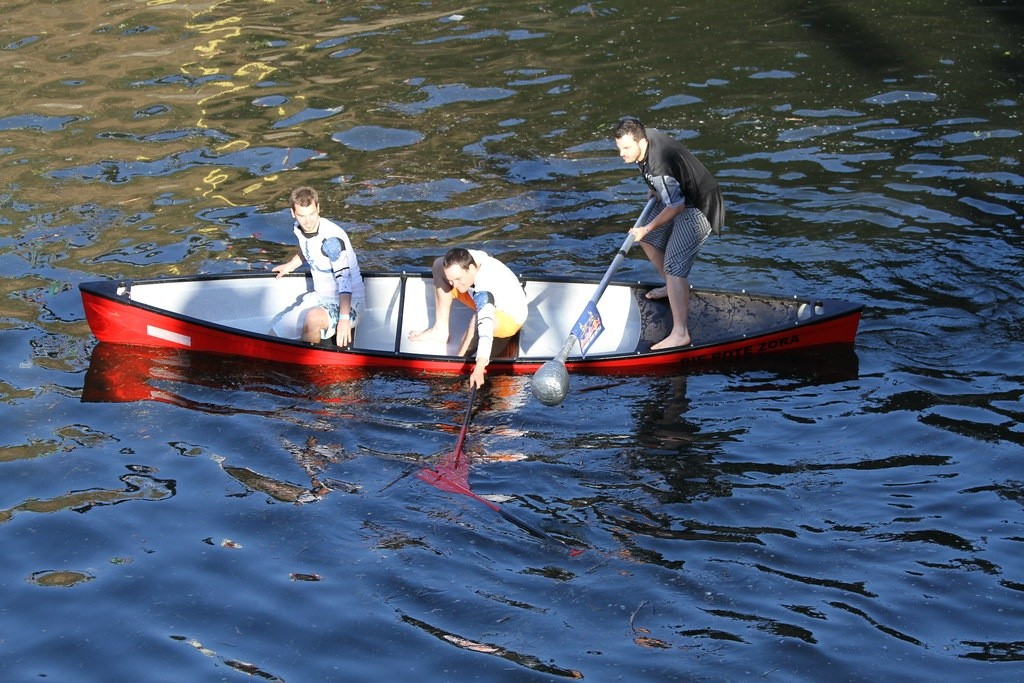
[644,226,650,234]
[338,315,350,320]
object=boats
[78,271,864,377]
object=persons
[614,119,727,349]
[409,247,530,388]
[267,187,366,347]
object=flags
[570,301,606,360]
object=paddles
[441,382,476,479]
[415,465,566,546]
